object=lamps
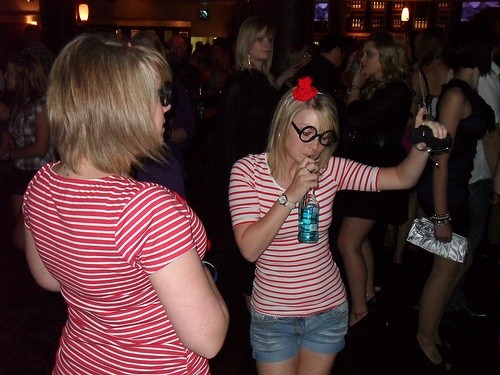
[79,3,88,22]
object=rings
[304,167,313,174]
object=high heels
[414,336,455,374]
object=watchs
[277,195,295,209]
[5,150,11,160]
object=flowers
[291,76,317,102]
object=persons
[0,13,500,375]
[21,33,230,374]
[227,75,448,375]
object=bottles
[297,188,319,244]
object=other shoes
[348,294,377,330]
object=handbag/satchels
[406,217,468,264]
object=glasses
[291,121,338,147]
[155,81,175,107]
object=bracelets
[202,260,218,283]
[350,85,361,91]
[432,213,451,226]
